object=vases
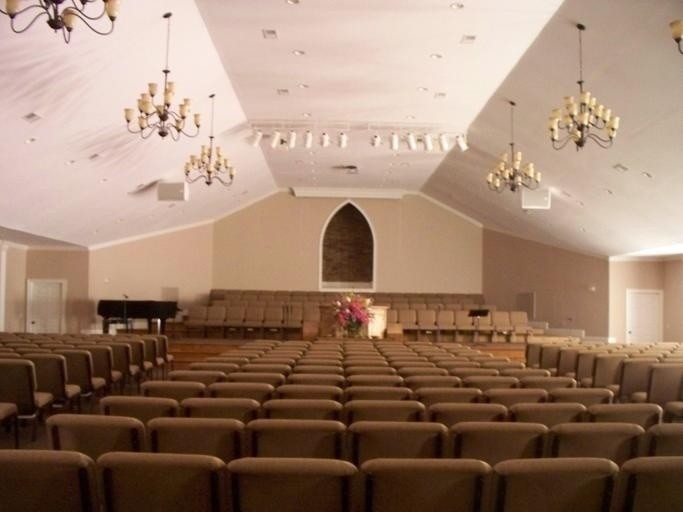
[348,320,361,337]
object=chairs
[550,424,645,466]
[207,358,249,367]
[0,450,96,512]
[415,388,484,408]
[359,459,492,512]
[588,404,663,431]
[240,364,292,378]
[46,415,145,459]
[261,400,342,421]
[227,373,286,388]
[512,312,533,341]
[526,343,542,367]
[41,345,74,349]
[185,308,206,338]
[292,365,346,379]
[461,377,519,394]
[404,376,461,392]
[346,376,404,388]
[437,361,480,370]
[77,345,124,394]
[428,403,509,430]
[250,359,295,369]
[474,311,493,343]
[53,350,105,410]
[0,403,19,450]
[297,360,342,367]
[131,337,164,381]
[531,344,569,369]
[23,354,81,414]
[450,369,501,381]
[168,371,227,388]
[276,385,343,404]
[398,311,418,340]
[115,341,153,385]
[344,360,388,368]
[245,310,264,338]
[265,309,283,338]
[630,355,663,359]
[146,418,244,463]
[140,382,207,404]
[225,309,244,338]
[346,367,398,379]
[437,311,456,342]
[286,374,346,391]
[1,343,3,348]
[188,363,239,375]
[664,402,682,422]
[548,348,586,374]
[0,349,16,352]
[209,289,497,310]
[520,377,577,393]
[245,420,348,457]
[387,310,399,324]
[549,388,614,408]
[494,458,618,512]
[304,302,320,338]
[227,459,359,512]
[16,349,52,353]
[659,358,681,363]
[344,387,415,405]
[502,370,551,380]
[612,457,683,512]
[606,359,659,396]
[391,362,436,371]
[5,343,38,347]
[565,351,607,379]
[217,341,509,360]
[0,359,53,442]
[283,310,302,339]
[180,399,261,425]
[398,367,449,378]
[143,336,174,375]
[0,333,141,346]
[207,383,275,405]
[510,403,589,429]
[456,311,473,343]
[99,396,181,424]
[483,362,524,370]
[492,312,514,342]
[581,355,629,387]
[632,364,681,403]
[96,343,140,393]
[484,389,548,408]
[528,342,682,358]
[96,454,227,512]
[0,353,21,359]
[348,422,450,468]
[646,424,682,458]
[343,400,427,427]
[419,311,437,341]
[207,308,225,337]
[450,422,550,467]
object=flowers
[332,292,373,337]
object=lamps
[185,94,234,186]
[305,131,311,149]
[487,101,541,193]
[424,134,433,149]
[548,24,618,151]
[322,134,329,147]
[408,131,416,151]
[391,131,397,149]
[1,1,119,44]
[272,130,281,148]
[372,135,382,148]
[126,13,200,141]
[289,131,295,149]
[341,132,347,148]
[669,19,683,55]
[440,133,448,150]
[252,130,263,146]
[457,137,468,152]
